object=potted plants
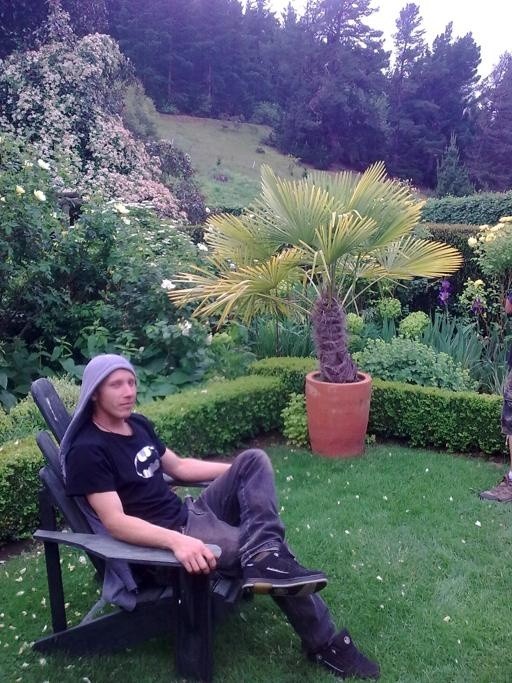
[165,158,465,455]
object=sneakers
[304,628,381,680]
[241,544,328,597]
[478,471,512,504]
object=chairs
[29,378,255,682]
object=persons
[477,291,512,502]
[55,352,385,679]
